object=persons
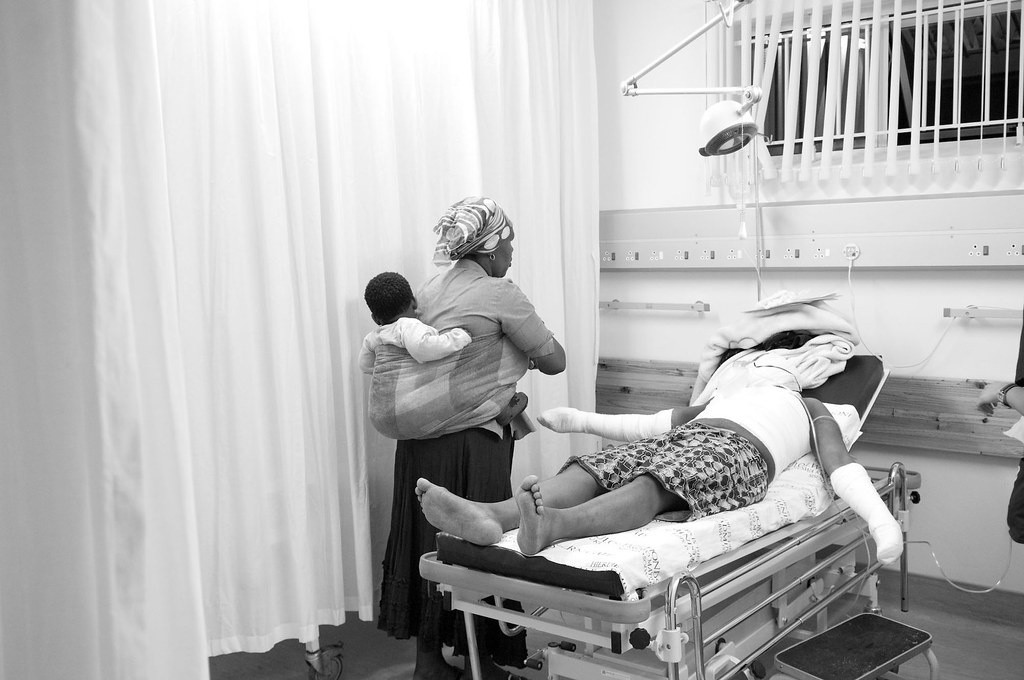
[377,192,567,679]
[357,270,528,442]
[975,308,1024,546]
[414,352,906,570]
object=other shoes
[500,671,527,680]
[443,666,464,680]
[496,392,528,425]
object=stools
[769,613,940,680]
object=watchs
[997,383,1018,408]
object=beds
[418,358,923,680]
